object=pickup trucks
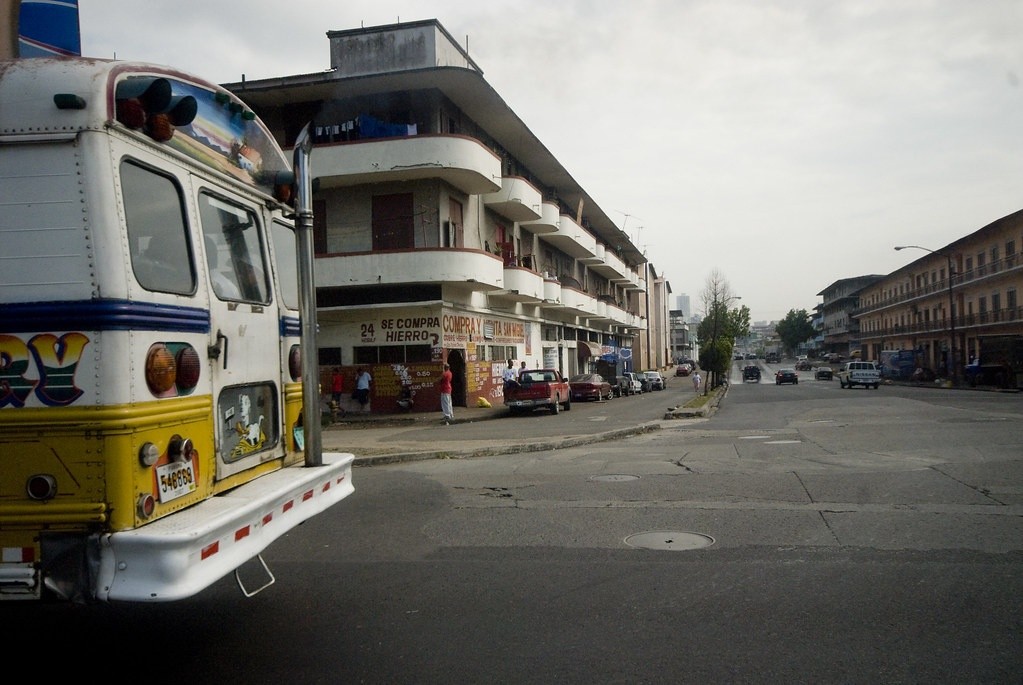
[503,369,570,414]
[840,362,881,389]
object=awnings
[577,341,602,357]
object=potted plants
[493,244,504,257]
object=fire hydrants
[330,400,340,424]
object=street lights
[894,246,958,389]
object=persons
[518,362,529,382]
[433,363,454,420]
[351,367,373,405]
[693,372,702,392]
[330,368,343,401]
[502,359,519,388]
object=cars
[775,369,798,385]
[612,371,663,397]
[676,363,693,376]
[568,374,614,401]
[815,367,833,381]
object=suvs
[741,366,761,383]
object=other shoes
[441,416,453,420]
